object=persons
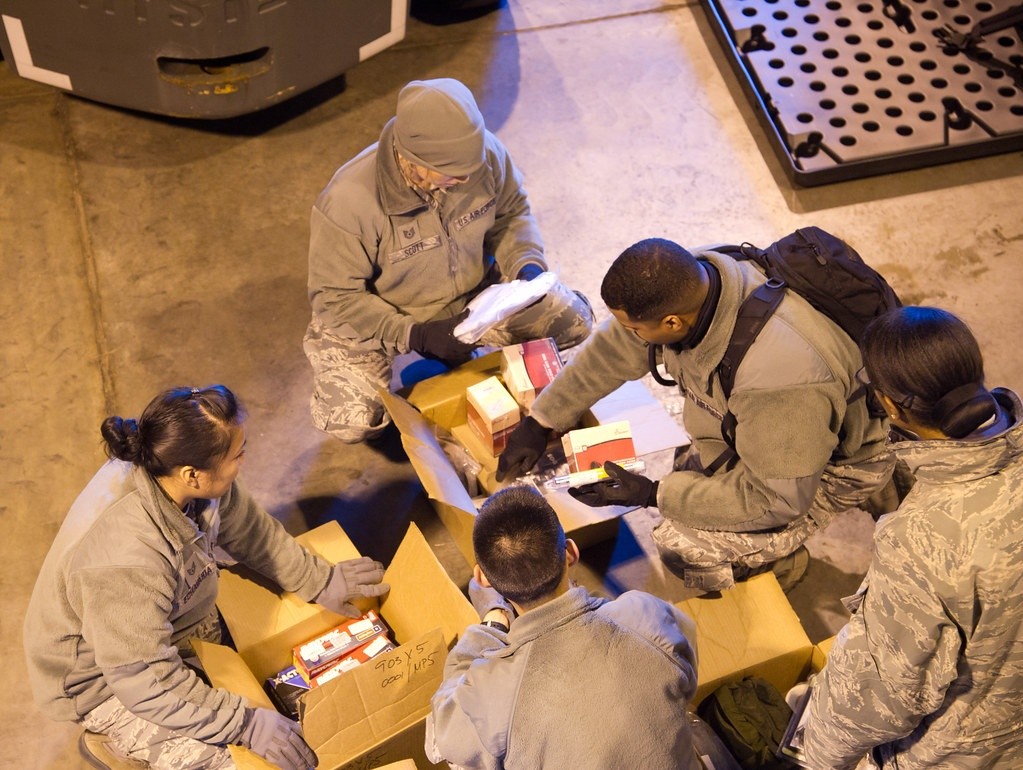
[496,239,894,594]
[25,385,392,769]
[302,78,597,444]
[803,306,1023,770]
[430,485,698,770]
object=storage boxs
[186,518,484,769]
[450,423,517,495]
[264,668,306,716]
[675,570,840,709]
[464,376,520,458]
[500,337,565,411]
[305,634,395,686]
[362,341,690,573]
[291,608,387,684]
[561,421,635,473]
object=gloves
[409,308,485,369]
[568,460,660,508]
[496,416,554,483]
[468,578,515,625]
[313,557,389,620]
[240,707,319,770]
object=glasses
[855,365,903,408]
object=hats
[393,78,486,177]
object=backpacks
[649,226,904,473]
[686,674,794,770]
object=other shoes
[772,544,810,595]
[79,729,149,770]
[862,459,916,522]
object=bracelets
[480,620,508,634]
